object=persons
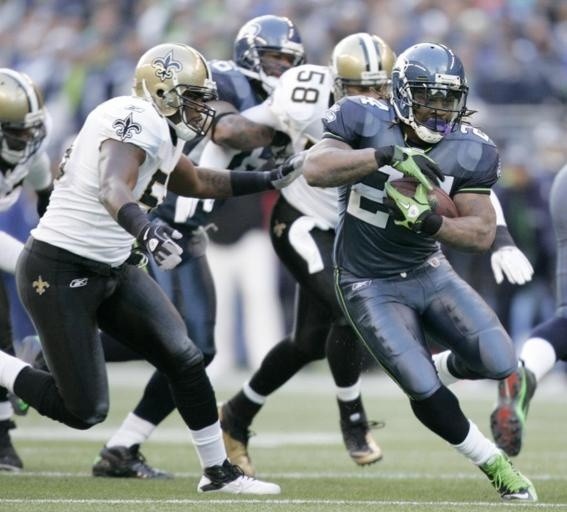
[203,191,284,407]
[175,31,536,479]
[0,66,54,473]
[491,158,567,458]
[301,43,540,504]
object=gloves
[381,182,440,237]
[490,245,535,285]
[138,218,183,273]
[270,149,308,190]
[387,143,445,192]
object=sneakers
[197,457,281,495]
[490,359,537,457]
[216,402,255,477]
[0,420,23,470]
[339,416,383,466]
[478,449,538,503]
[8,334,41,416]
[91,442,176,480]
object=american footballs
[385,175,459,222]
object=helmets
[232,14,306,95]
[0,67,46,165]
[327,32,397,105]
[392,42,469,142]
[131,43,218,141]
